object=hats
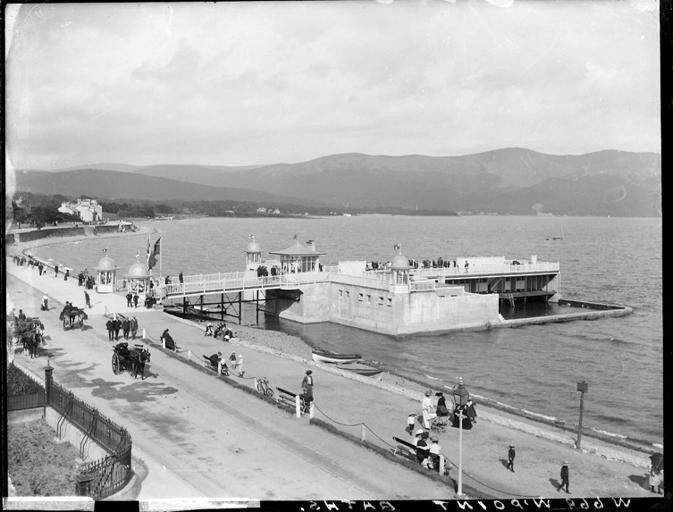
[430,435,438,441]
[416,429,423,434]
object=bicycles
[257,378,274,399]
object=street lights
[453,376,469,495]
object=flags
[146,237,161,271]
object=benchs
[160,336,178,353]
[202,354,224,374]
[392,435,450,476]
[276,385,309,416]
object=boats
[194,305,226,313]
[312,350,362,363]
[334,365,384,376]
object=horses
[67,302,76,327]
[129,348,151,379]
[23,324,44,359]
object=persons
[257,262,323,284]
[512,258,520,265]
[365,256,469,272]
[507,444,515,472]
[649,452,664,495]
[407,389,477,476]
[557,461,571,494]
[6,254,244,378]
[301,370,314,394]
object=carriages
[10,319,43,359]
[63,303,84,330]
[111,343,150,381]
